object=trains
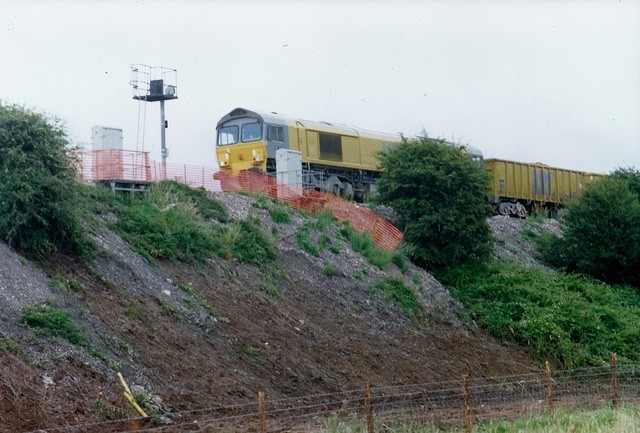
[216,106,610,217]
